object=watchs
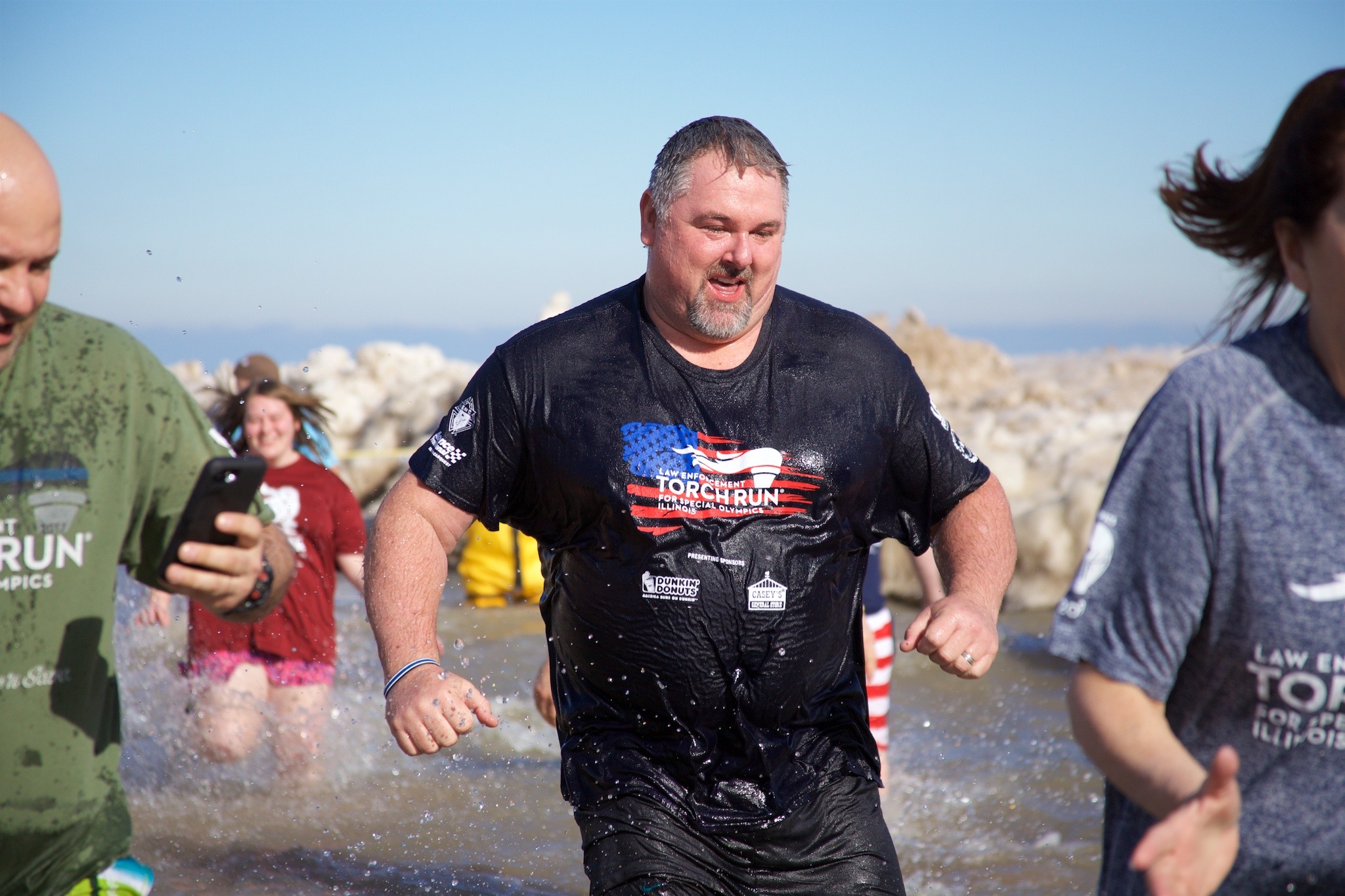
[221,559,273,617]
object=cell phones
[156,454,265,578]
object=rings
[962,651,975,665]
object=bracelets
[384,658,443,696]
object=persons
[457,521,544,608]
[137,369,443,776]
[1052,65,1345,896]
[363,115,1018,896]
[231,355,339,473]
[862,540,944,801]
[0,113,300,896]
